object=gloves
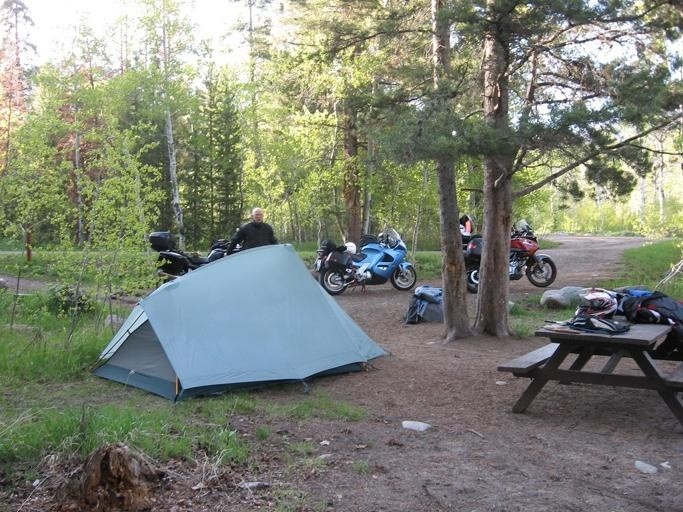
[568,315,630,335]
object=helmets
[344,242,356,254]
[574,287,618,319]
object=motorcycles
[459,214,557,294]
[149,228,242,287]
[314,227,417,296]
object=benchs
[497,343,683,393]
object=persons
[459,212,475,243]
[225,207,279,256]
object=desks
[512,316,682,426]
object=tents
[89,241,393,401]
